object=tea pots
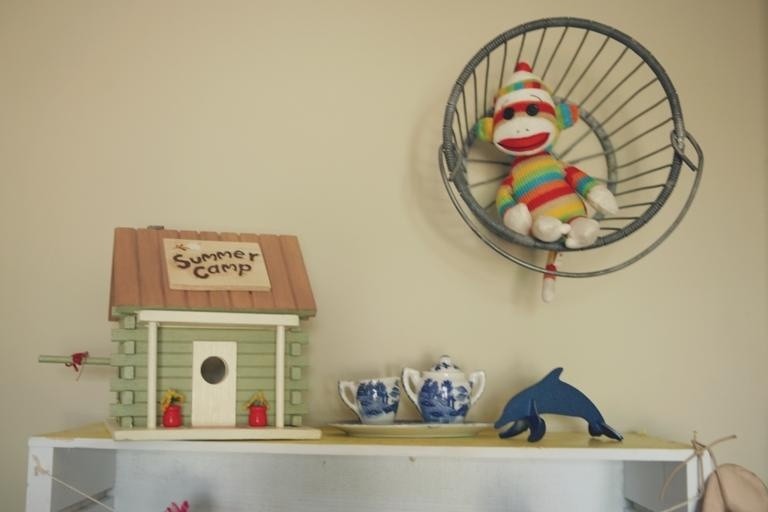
[402,353,487,425]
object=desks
[24,422,717,512]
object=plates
[328,421,496,439]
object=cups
[337,375,402,425]
